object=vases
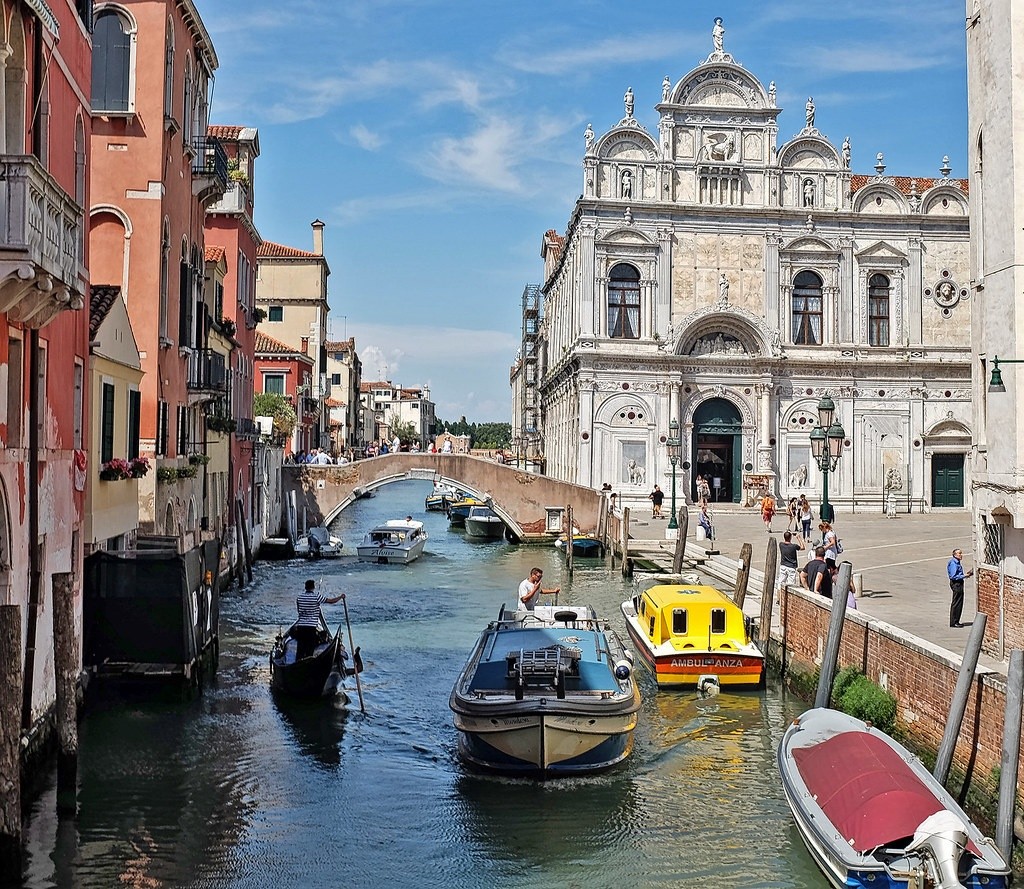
[101,472,115,481]
[131,471,142,478]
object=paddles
[341,595,364,708]
[554,592,558,606]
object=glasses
[534,573,542,579]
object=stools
[696,523,706,541]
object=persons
[297,580,346,659]
[776,532,805,605]
[601,483,617,509]
[786,494,814,543]
[799,546,830,595]
[517,568,559,611]
[946,549,970,627]
[285,433,452,464]
[495,449,504,463]
[649,484,665,520]
[820,500,834,545]
[832,561,857,610]
[696,475,710,507]
[808,539,821,560]
[698,505,715,541]
[817,522,838,567]
[404,516,415,539]
[761,493,776,533]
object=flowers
[127,457,152,478]
[104,458,129,474]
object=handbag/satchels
[786,507,796,516]
[432,448,437,453]
[835,536,844,554]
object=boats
[558,533,602,557]
[777,708,1013,889]
[425,484,459,510]
[464,506,506,538]
[356,520,429,564]
[620,572,766,696]
[271,607,363,698]
[448,603,642,772]
[260,535,291,553]
[447,491,487,523]
[294,527,343,558]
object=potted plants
[157,464,178,486]
[249,307,267,328]
[189,455,212,465]
[227,158,249,190]
[176,464,198,480]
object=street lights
[521,436,529,470]
[808,392,845,544]
[665,417,681,540]
[514,436,521,469]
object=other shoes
[807,537,810,543]
[652,516,656,519]
[766,528,773,533]
[776,600,779,605]
[952,623,964,628]
[802,540,806,543]
